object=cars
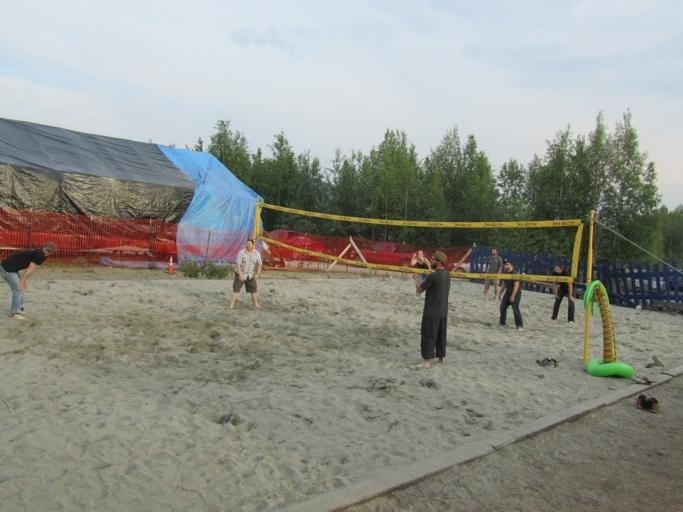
[620,269,663,292]
[451,256,487,274]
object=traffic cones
[165,254,173,273]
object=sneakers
[646,397,660,413]
[636,394,647,410]
[537,358,556,367]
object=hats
[47,242,56,253]
[431,250,448,264]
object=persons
[497,262,523,330]
[411,250,451,368]
[0,242,56,320]
[229,240,263,309]
[548,263,575,322]
[483,248,504,299]
[410,250,432,296]
